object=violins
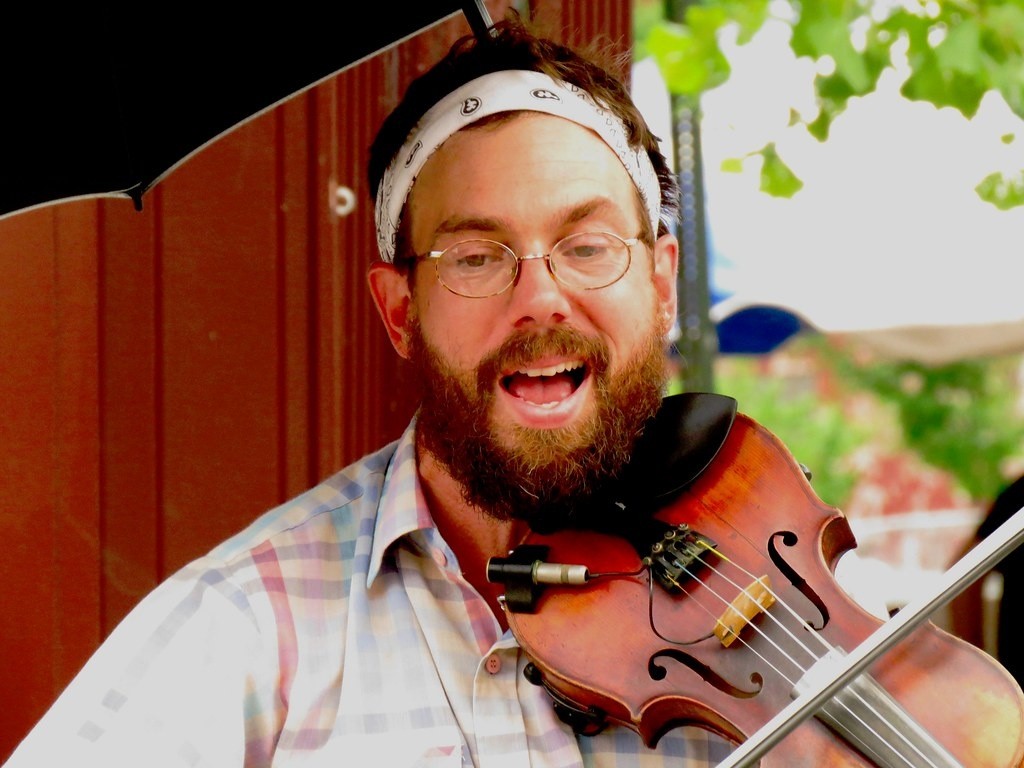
[485,391,1024,768]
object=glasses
[415,231,638,298]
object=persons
[1,19,892,768]
